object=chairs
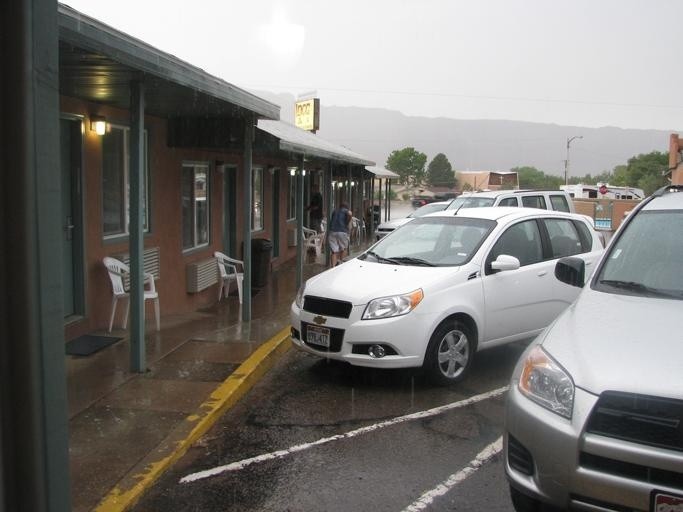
[302,216,369,261]
[102,257,160,331]
[213,251,245,305]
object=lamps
[90,115,108,135]
[289,163,298,176]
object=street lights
[565,134,583,185]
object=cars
[286,203,605,382]
[375,190,575,241]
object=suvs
[497,182,681,510]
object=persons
[303,184,323,235]
[328,202,353,267]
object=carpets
[65,334,123,355]
[229,288,261,298]
